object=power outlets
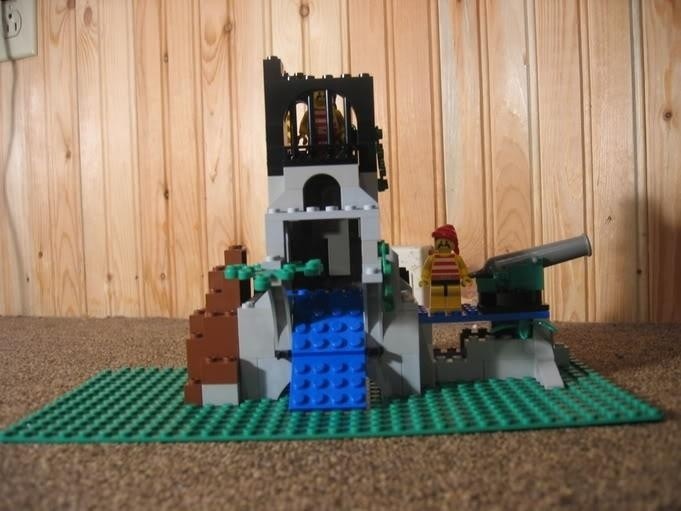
[0,0,38,61]
